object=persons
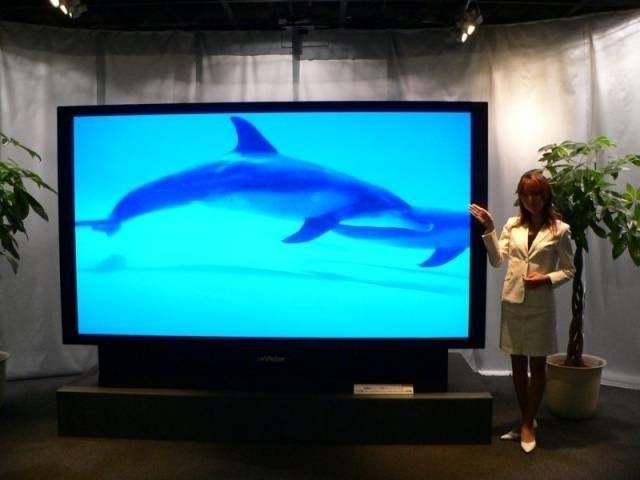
[469,172,577,453]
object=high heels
[499,419,538,453]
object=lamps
[49,0,89,19]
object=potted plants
[0,131,60,406]
[511,134,640,422]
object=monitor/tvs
[57,101,489,389]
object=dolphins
[75,116,434,244]
[329,206,471,268]
